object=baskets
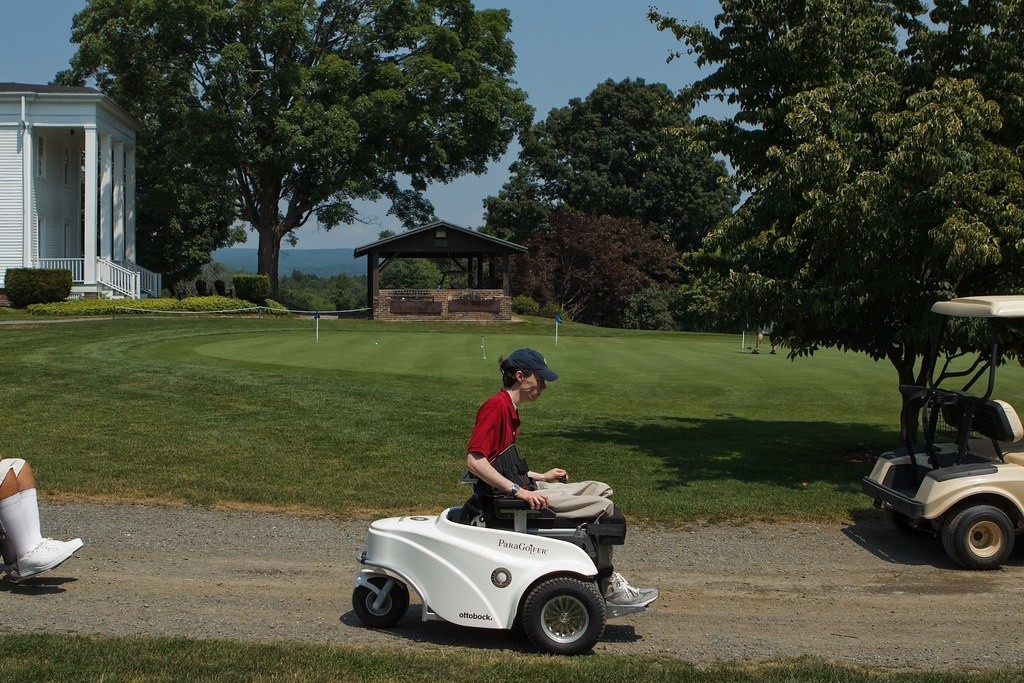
[929,409,982,439]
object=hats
[505,348,559,382]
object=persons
[752,322,777,354]
[0,455,84,578]
[465,348,660,608]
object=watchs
[510,484,520,496]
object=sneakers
[17,538,83,579]
[601,573,658,607]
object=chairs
[942,392,1024,467]
[458,471,556,538]
